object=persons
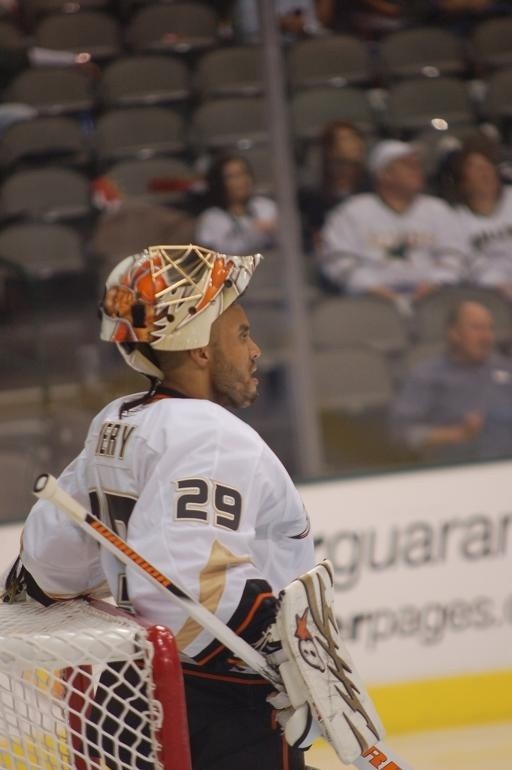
[229,1,506,47]
[21,244,323,770]
[197,154,277,258]
[392,293,511,464]
[296,120,512,314]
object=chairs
[0,0,512,518]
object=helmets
[97,244,263,352]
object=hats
[365,138,425,175]
